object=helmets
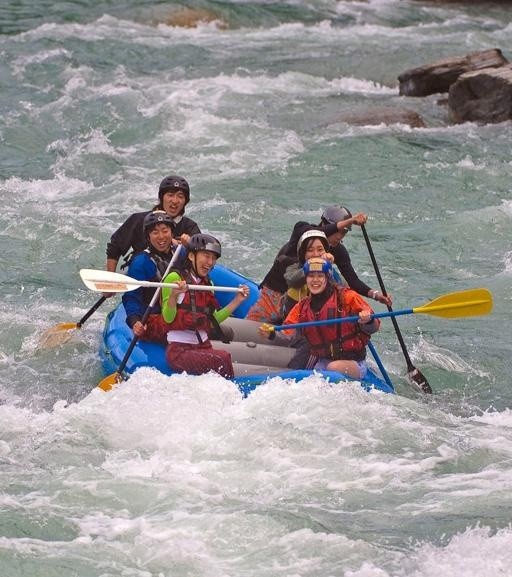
[143,211,174,232]
[304,258,333,278]
[297,230,329,259]
[324,206,352,231]
[159,176,189,192]
[188,234,221,258]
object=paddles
[97,236,183,393]
[79,269,243,292]
[360,223,432,394]
[260,288,493,331]
[58,295,107,330]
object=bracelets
[372,291,380,299]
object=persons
[259,257,380,380]
[245,204,393,323]
[161,234,250,378]
[121,211,234,341]
[103,176,200,298]
[281,230,351,319]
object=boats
[97,262,395,395]
